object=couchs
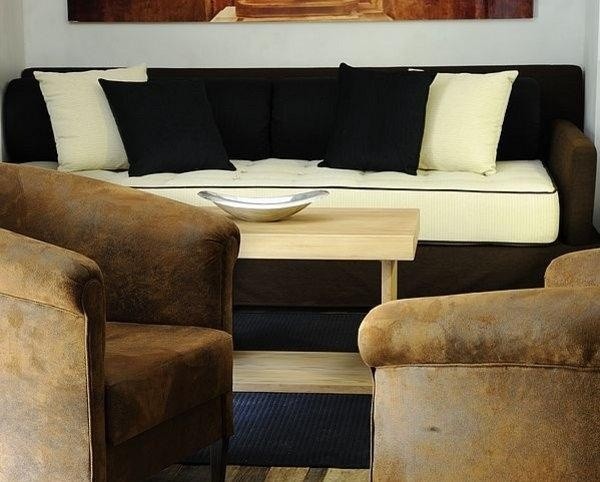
[0,65,600,307]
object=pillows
[319,63,437,179]
[32,66,149,171]
[408,68,520,175]
[97,78,237,178]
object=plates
[197,189,330,222]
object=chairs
[1,162,240,482]
[357,247,600,482]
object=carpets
[173,391,373,470]
[232,307,367,352]
